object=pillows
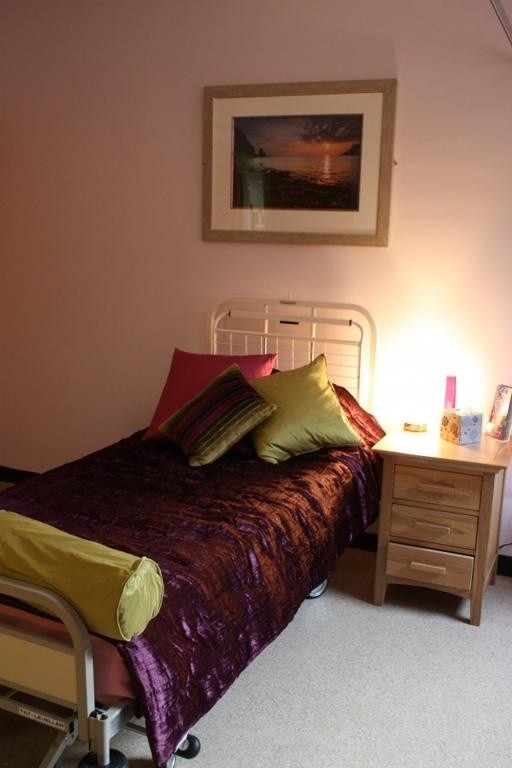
[249,353,368,467]
[144,347,280,443]
[159,363,279,468]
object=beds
[0,295,379,768]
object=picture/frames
[201,77,399,247]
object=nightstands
[370,427,512,627]
